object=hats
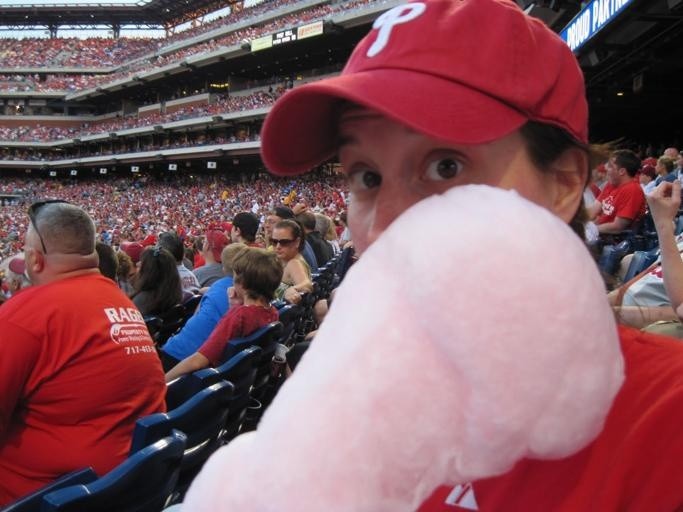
[118,241,145,263]
[7,258,31,282]
[257,1,590,177]
[206,229,229,263]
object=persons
[1,0,682,510]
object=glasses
[269,238,295,246]
[153,245,162,273]
[26,199,69,256]
